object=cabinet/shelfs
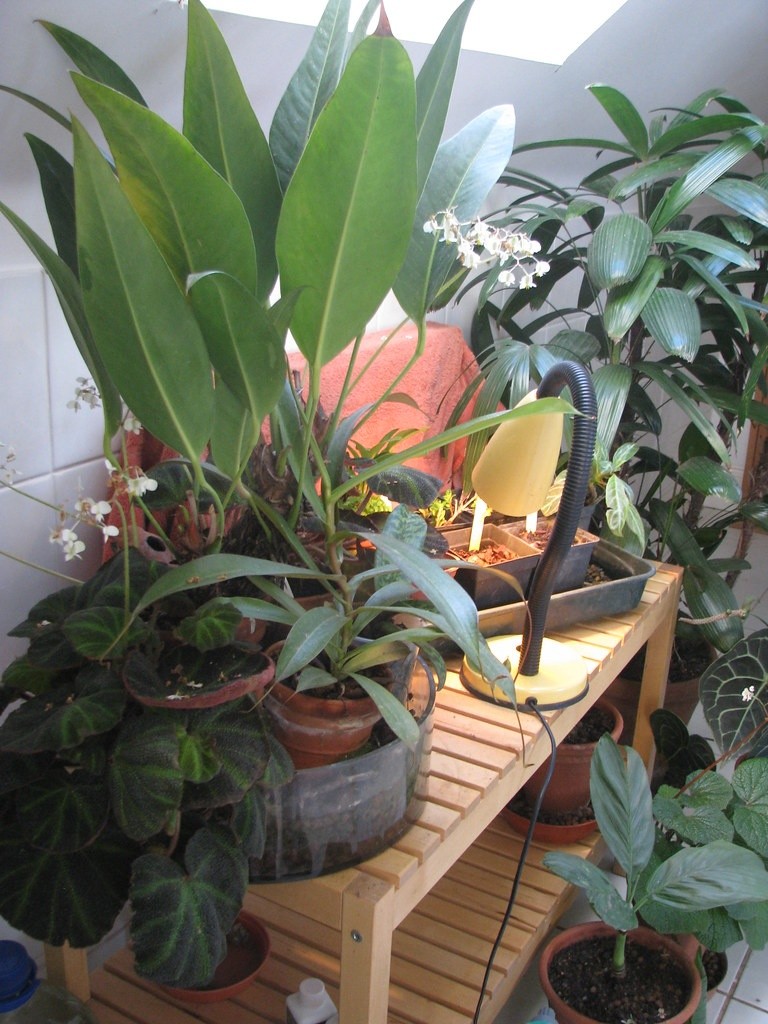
[46,553,686,1024]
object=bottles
[284,977,339,1024]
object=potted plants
[0,0,768,996]
[538,734,767,1024]
